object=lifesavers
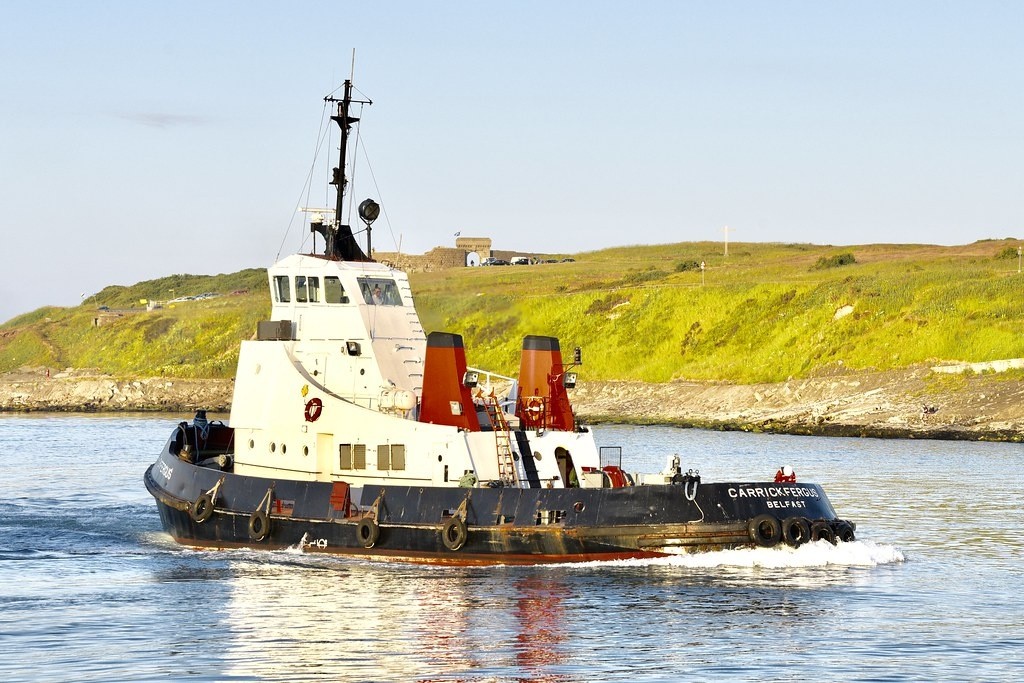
[304,398,325,421]
[525,394,545,422]
[356,516,378,549]
[191,493,214,525]
[748,513,855,547]
[442,517,467,551]
[247,510,270,543]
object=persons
[371,288,385,305]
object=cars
[479,256,578,266]
[167,289,251,305]
[97,305,109,311]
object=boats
[143,47,857,566]
